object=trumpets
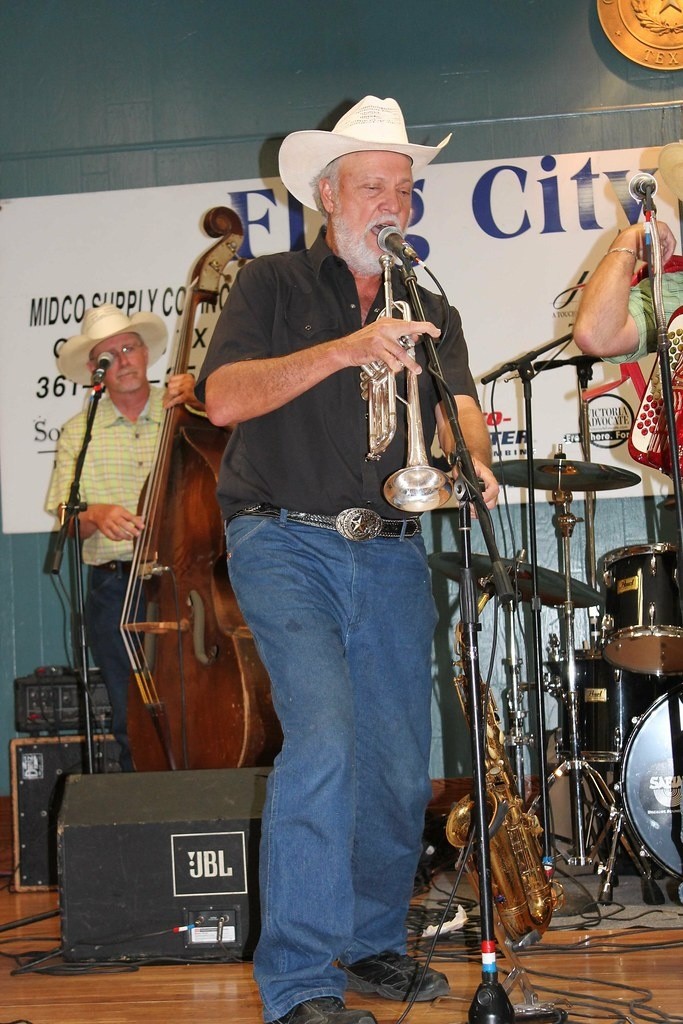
[360,252,454,514]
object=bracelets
[605,247,638,260]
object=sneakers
[338,949,450,1002]
[268,996,378,1024]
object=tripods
[525,471,668,908]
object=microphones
[629,172,657,201]
[377,226,419,263]
[94,351,113,385]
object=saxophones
[443,618,554,941]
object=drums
[555,650,683,762]
[596,543,683,674]
[617,684,683,879]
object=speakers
[52,765,276,961]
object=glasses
[91,340,145,363]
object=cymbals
[481,458,641,492]
[425,551,603,609]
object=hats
[55,301,168,385]
[658,142,683,201]
[279,95,452,211]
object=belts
[225,502,422,542]
[94,558,133,573]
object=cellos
[118,207,284,774]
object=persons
[44,305,206,773]
[194,94,499,1024]
[572,220,683,906]
